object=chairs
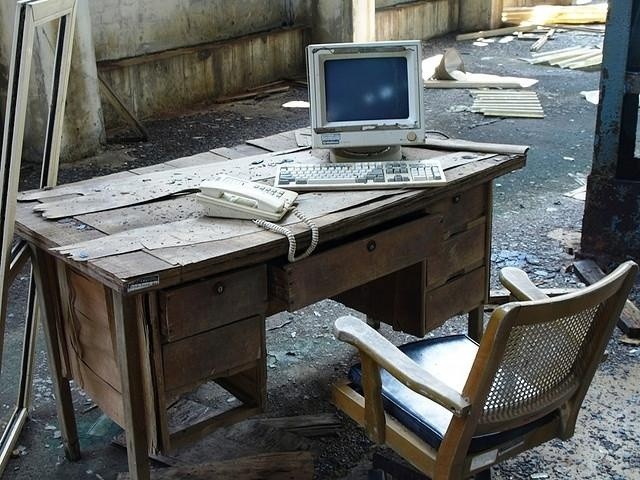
[321,259,639,480]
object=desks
[15,123,527,480]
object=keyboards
[275,158,448,191]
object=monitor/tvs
[306,40,426,162]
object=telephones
[195,174,299,223]
